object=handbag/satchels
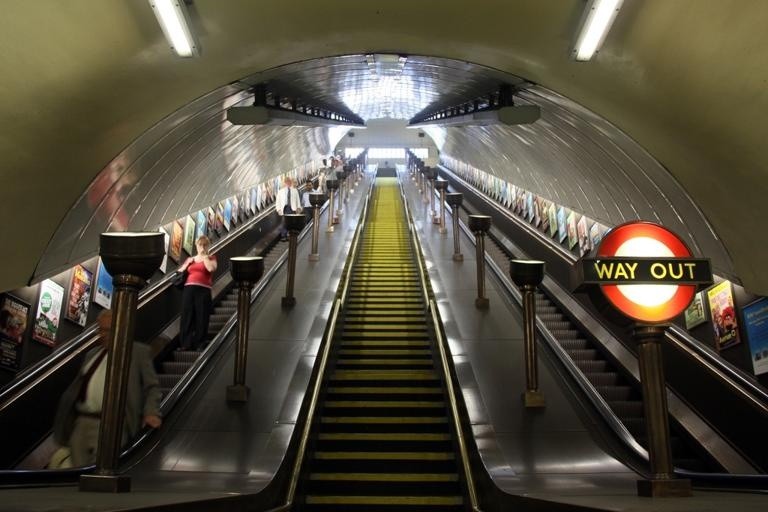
[174,268,188,290]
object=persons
[274,147,351,242]
[710,301,740,345]
[51,310,165,469]
[46,318,58,332]
[77,287,90,325]
[177,235,218,350]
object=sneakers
[281,236,287,242]
[286,236,290,241]
[197,343,206,352]
[177,345,194,351]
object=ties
[77,345,109,404]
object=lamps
[404,84,544,131]
[567,0,627,69]
[144,0,204,66]
[222,85,368,136]
